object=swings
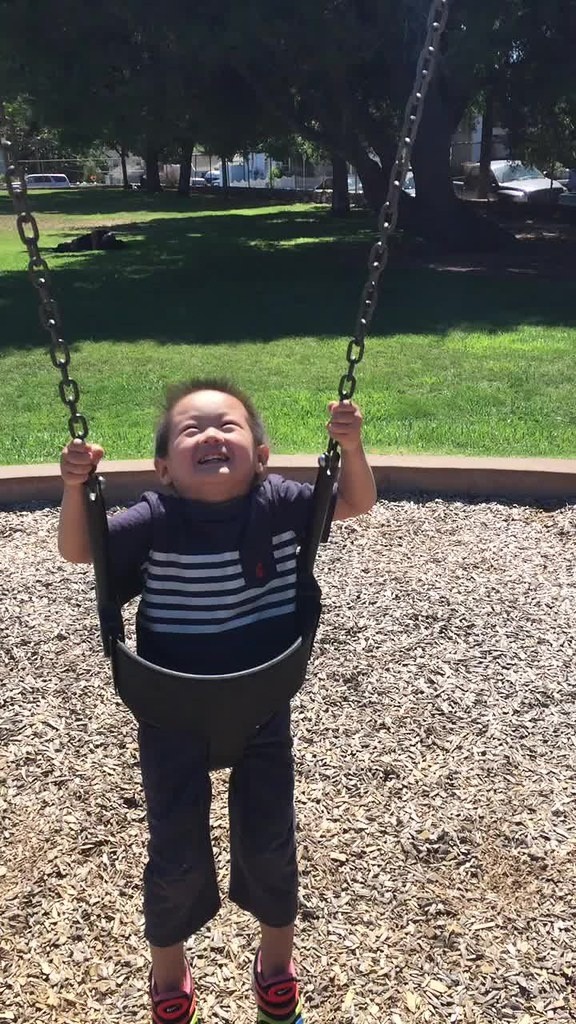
[0,0,453,771]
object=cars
[190,177,207,189]
[10,182,21,190]
[313,177,355,195]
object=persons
[50,228,127,252]
[56,374,377,1024]
[138,175,147,187]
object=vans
[17,174,71,190]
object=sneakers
[149,956,197,1024]
[251,945,303,1024]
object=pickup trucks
[349,160,576,206]
